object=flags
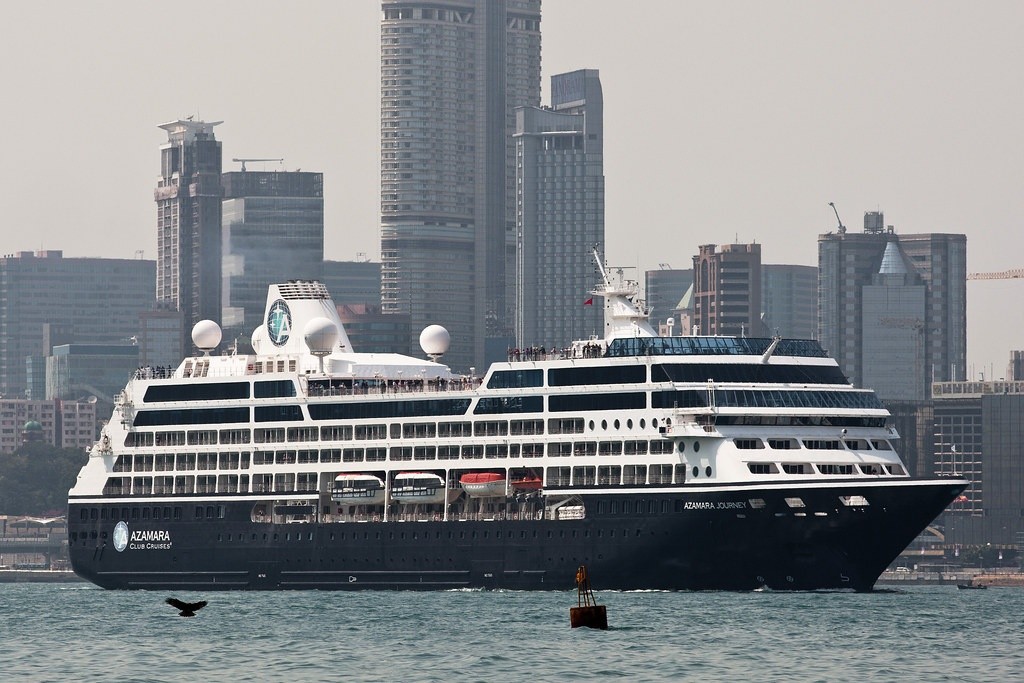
[585,299,593,307]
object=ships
[63,283,972,597]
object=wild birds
[166,597,207,618]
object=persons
[621,337,712,361]
[306,376,483,396]
[135,364,172,380]
[505,342,636,362]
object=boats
[390,472,464,505]
[456,472,516,498]
[957,585,987,590]
[327,472,386,509]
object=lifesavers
[248,364,253,370]
